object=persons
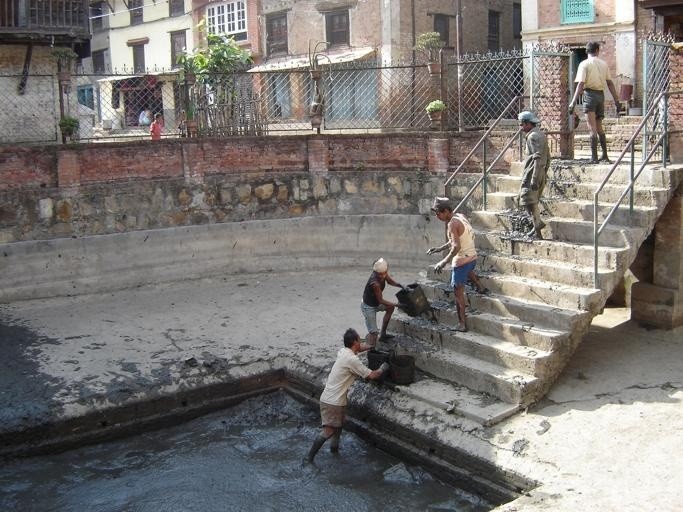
[360,258,406,345]
[568,41,622,164]
[517,111,550,240]
[426,197,488,331]
[303,328,389,464]
[150,113,164,141]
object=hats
[518,111,541,124]
[431,196,450,214]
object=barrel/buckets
[367,346,394,378]
[389,355,415,385]
[395,282,430,317]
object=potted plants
[59,117,78,137]
[425,99,446,120]
[308,37,332,81]
[412,31,447,73]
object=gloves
[380,362,390,372]
